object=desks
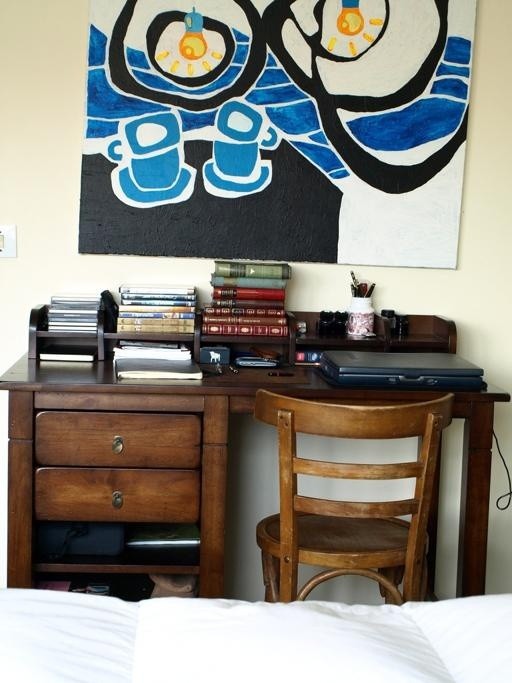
[0,358,512,598]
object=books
[113,341,203,379]
[45,297,100,332]
[38,344,97,362]
[127,525,201,545]
[202,261,292,337]
[294,350,324,367]
[116,286,196,334]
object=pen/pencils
[350,284,358,298]
[268,370,296,376]
[214,360,225,377]
[350,271,361,288]
[228,364,241,376]
[364,282,376,299]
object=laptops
[317,350,484,390]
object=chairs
[254,388,454,600]
